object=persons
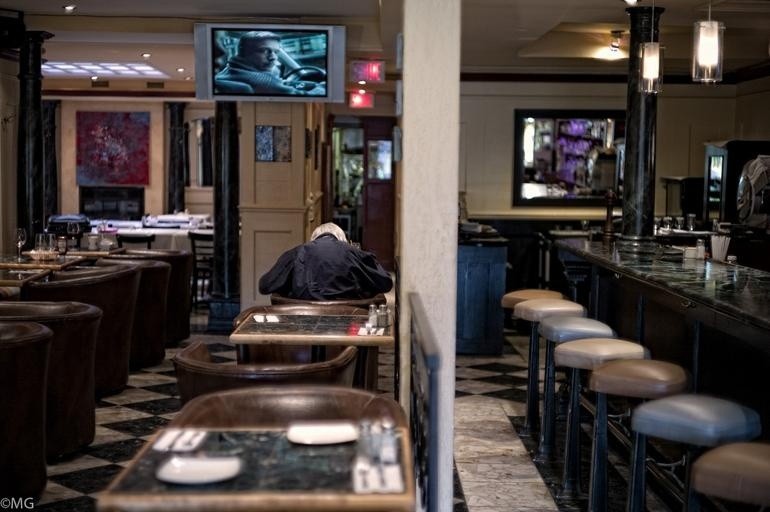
[214,32,301,95]
[259,221,394,302]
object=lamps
[693,0,724,83]
[638,0,663,94]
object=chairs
[188,231,214,312]
[233,304,369,329]
[270,294,387,310]
[0,250,191,512]
[173,340,360,409]
[168,385,408,426]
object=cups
[683,259,696,269]
[654,213,697,234]
[681,247,697,260]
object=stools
[500,287,769,512]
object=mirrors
[512,108,626,207]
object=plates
[157,456,243,485]
[287,422,361,448]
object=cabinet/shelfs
[704,140,769,231]
[458,207,622,356]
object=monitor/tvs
[193,22,345,103]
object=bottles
[376,416,403,462]
[368,303,378,327]
[375,305,389,326]
[727,255,737,264]
[361,418,379,460]
[696,239,707,261]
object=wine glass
[10,219,116,269]
